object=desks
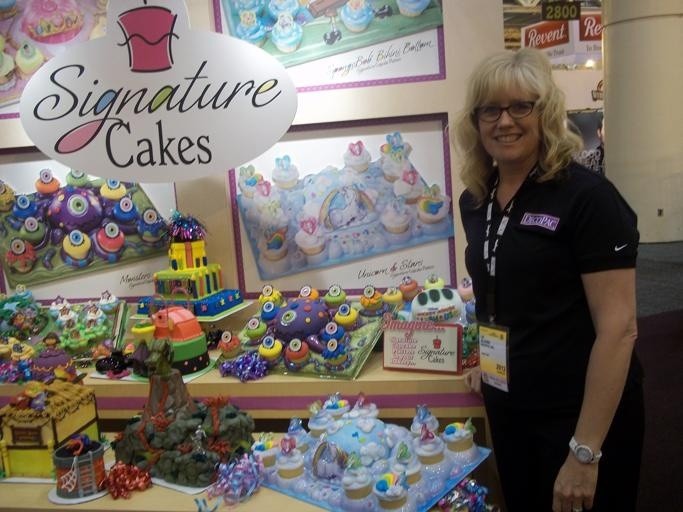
[0,301,498,511]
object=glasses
[474,101,535,123]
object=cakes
[226,1,431,53]
[0,0,106,91]
[0,209,482,512]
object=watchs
[568,434,602,465]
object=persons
[42,332,60,353]
[448,46,646,512]
[580,114,604,173]
